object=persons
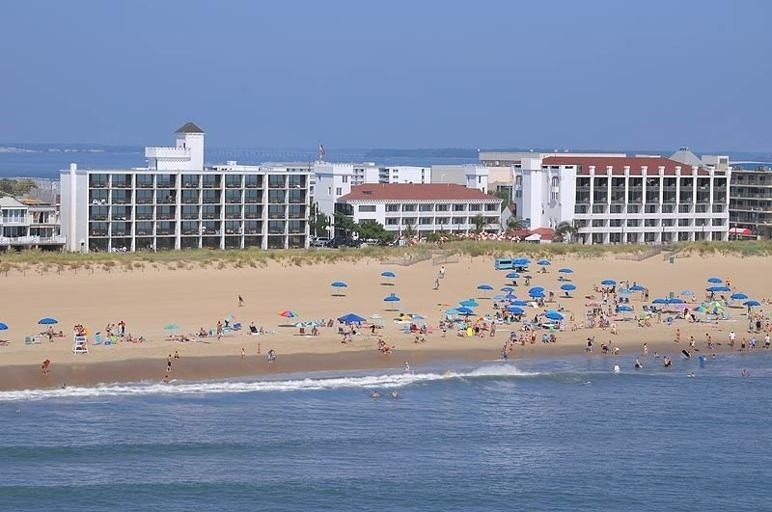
[106,319,141,344]
[267,349,274,368]
[240,346,246,359]
[48,326,63,337]
[371,389,398,399]
[39,360,50,375]
[163,351,180,383]
[341,320,496,370]
[75,324,86,353]
[199,321,224,341]
[472,278,771,376]
[311,326,320,336]
[439,265,447,278]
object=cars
[309,235,363,248]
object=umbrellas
[512,258,551,266]
[0,322,8,330]
[38,317,58,324]
